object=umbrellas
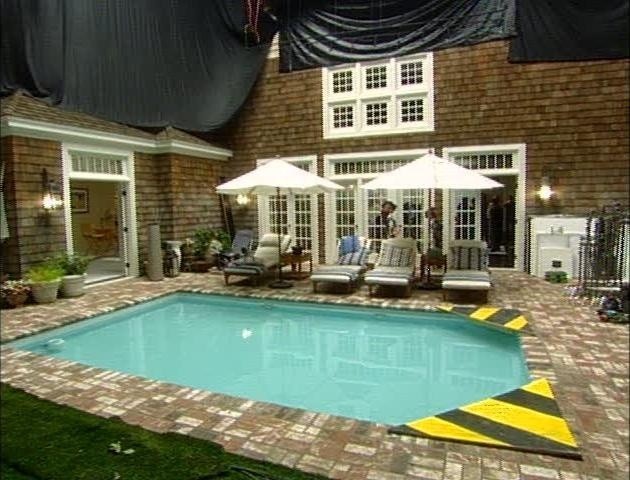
[361,151,505,282]
[214,154,346,284]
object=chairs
[309,237,417,299]
[80,223,117,257]
[216,229,292,287]
[441,238,492,304]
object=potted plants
[1,249,95,307]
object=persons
[371,194,515,256]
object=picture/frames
[69,187,91,214]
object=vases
[146,222,183,282]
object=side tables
[420,253,447,284]
[281,252,312,280]
